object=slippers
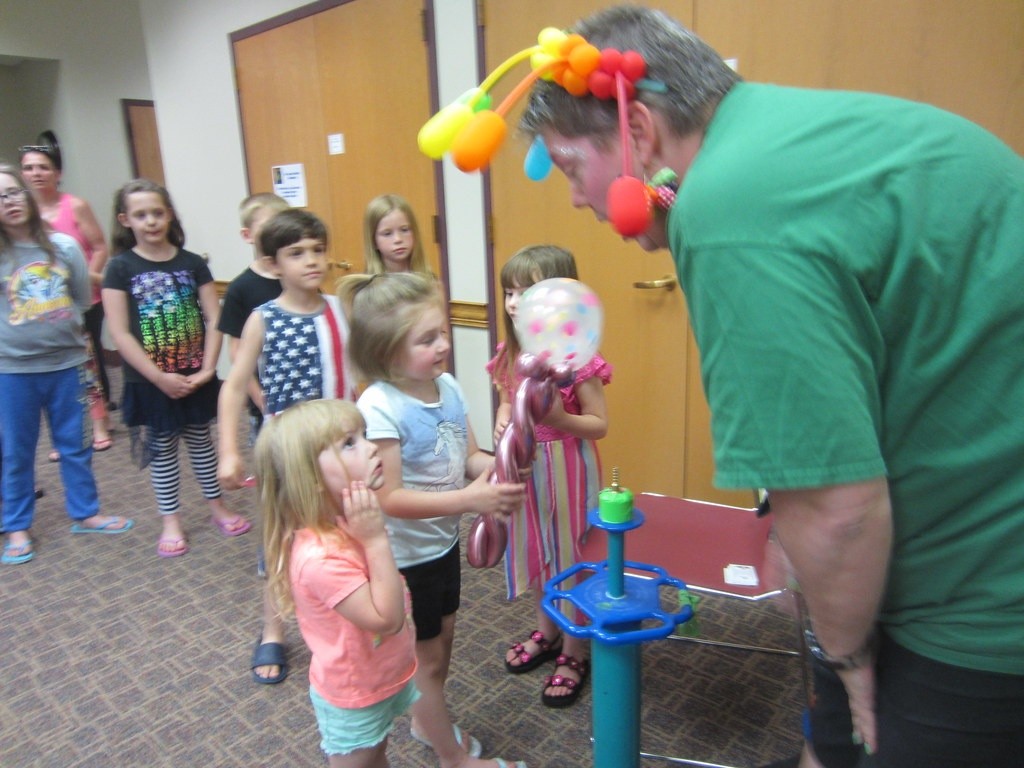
[491,757,528,768]
[157,534,187,556]
[211,514,251,537]
[1,539,36,565]
[410,724,482,759]
[250,627,289,685]
[71,516,132,535]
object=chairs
[575,489,825,768]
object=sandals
[505,630,564,674]
[542,653,589,708]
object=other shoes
[48,400,119,462]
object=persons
[216,190,528,768]
[521,8,1024,768]
[253,398,422,768]
[101,177,252,558]
[486,243,614,708]
[0,129,133,565]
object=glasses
[0,189,30,203]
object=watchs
[804,618,876,671]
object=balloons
[417,24,652,239]
[465,278,605,567]
